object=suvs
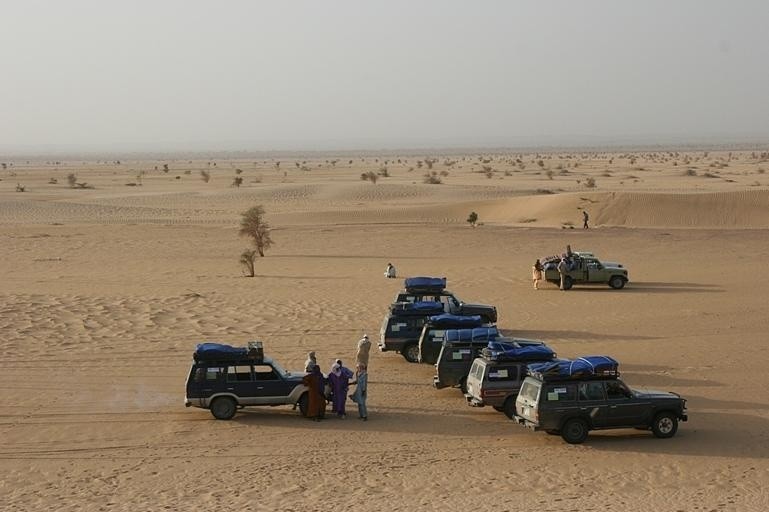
[534,248,636,289]
[181,341,339,422]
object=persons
[581,212,589,228]
[355,333,372,371]
[557,258,568,290]
[304,351,368,423]
[383,262,396,278]
[533,259,543,289]
